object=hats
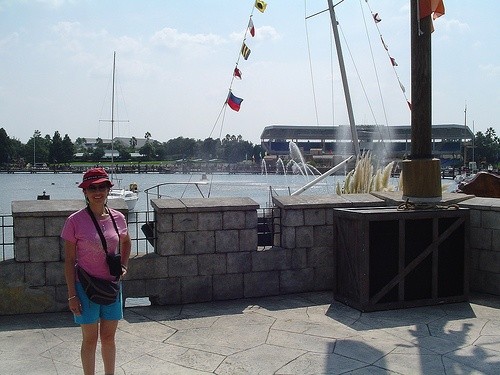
[78,168,114,188]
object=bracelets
[120,264,128,272]
[67,295,80,301]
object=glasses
[86,184,107,192]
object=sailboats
[21,134,50,172]
[453,103,477,183]
[97,51,139,220]
[143,0,411,246]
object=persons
[6,161,286,174]
[60,166,131,375]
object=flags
[233,68,242,80]
[417,0,445,35]
[255,0,267,13]
[248,18,255,37]
[372,12,381,24]
[241,42,251,60]
[390,58,398,67]
[224,90,244,111]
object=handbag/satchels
[76,264,121,306]
[108,254,122,276]
